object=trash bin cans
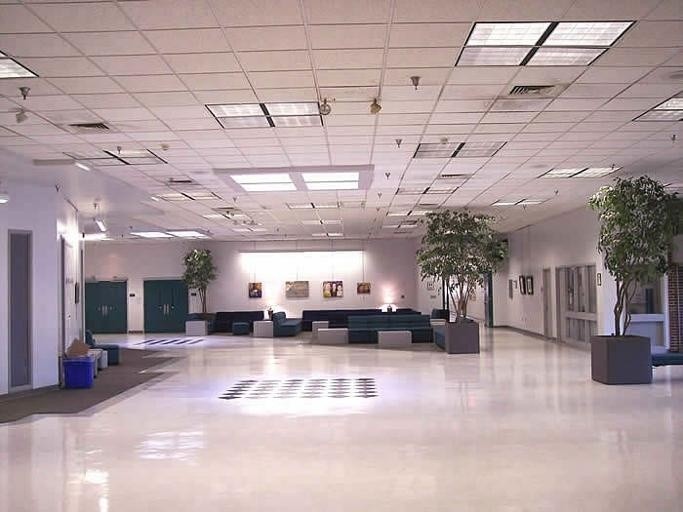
[102,344,119,368]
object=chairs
[85,329,119,366]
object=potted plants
[582,173,683,386]
[414,208,510,355]
[182,248,215,334]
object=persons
[336,284,342,296]
[248,283,261,298]
[323,283,331,297]
[329,283,336,296]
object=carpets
[1,347,185,425]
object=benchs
[184,307,479,354]
[79,349,103,377]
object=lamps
[95,203,107,233]
[368,98,381,114]
[318,97,330,115]
[0,193,10,205]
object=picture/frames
[518,275,525,296]
[526,275,533,295]
